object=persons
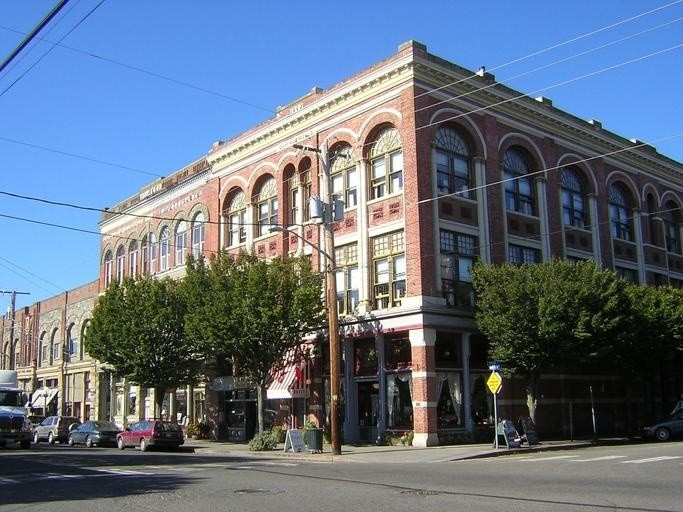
[208,416,219,442]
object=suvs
[28,416,81,447]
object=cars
[67,421,124,447]
[115,419,184,452]
[638,398,682,441]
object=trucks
[0,369,33,450]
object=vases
[405,442,408,446]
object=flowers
[401,429,414,442]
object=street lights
[267,226,342,453]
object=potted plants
[188,423,197,440]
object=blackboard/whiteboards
[501,416,539,448]
[288,430,305,450]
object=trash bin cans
[306,428,323,454]
